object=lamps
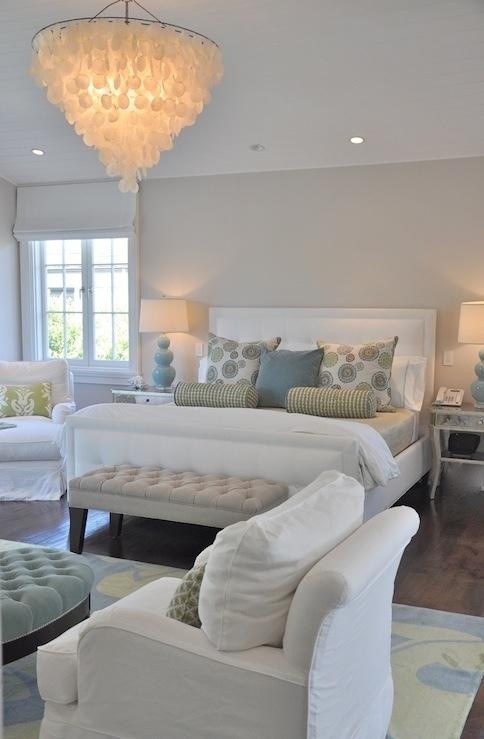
[457,302,484,409]
[138,298,188,389]
[31,1,223,195]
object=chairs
[0,360,75,502]
[34,469,419,739]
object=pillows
[173,332,399,416]
[166,465,365,653]
[0,382,53,420]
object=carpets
[0,538,484,739]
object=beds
[63,308,441,527]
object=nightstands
[110,386,173,406]
[428,404,483,500]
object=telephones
[435,386,464,407]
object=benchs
[68,464,287,555]
